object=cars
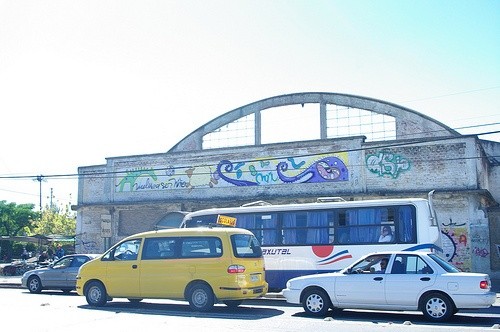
[282,251,496,323]
[22,254,105,294]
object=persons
[378,226,394,242]
[21,244,66,263]
[354,258,389,274]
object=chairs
[148,243,177,257]
[394,260,405,273]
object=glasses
[383,229,389,232]
[380,262,386,265]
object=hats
[56,243,62,248]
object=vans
[75,227,268,313]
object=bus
[177,197,444,293]
